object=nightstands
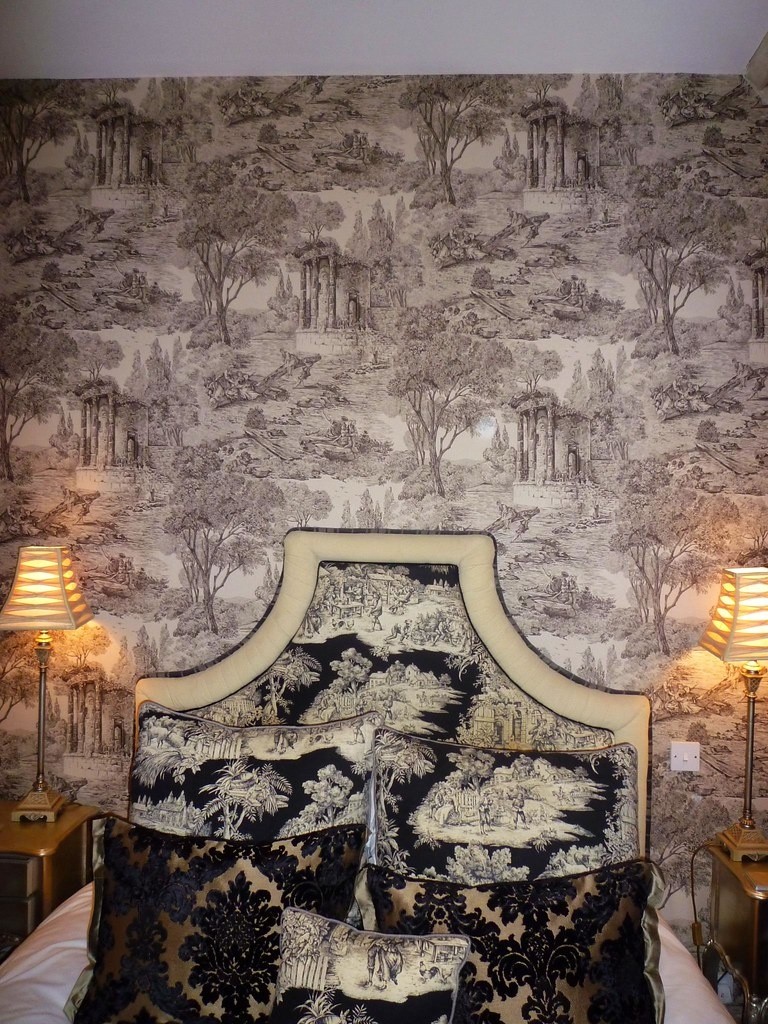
[0,801,101,946]
[705,847,768,1024]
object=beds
[0,525,739,1024]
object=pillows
[64,812,374,1024]
[371,724,640,886]
[126,699,385,931]
[353,857,666,1024]
[269,904,470,1024]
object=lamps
[0,545,96,823]
[690,566,768,970]
